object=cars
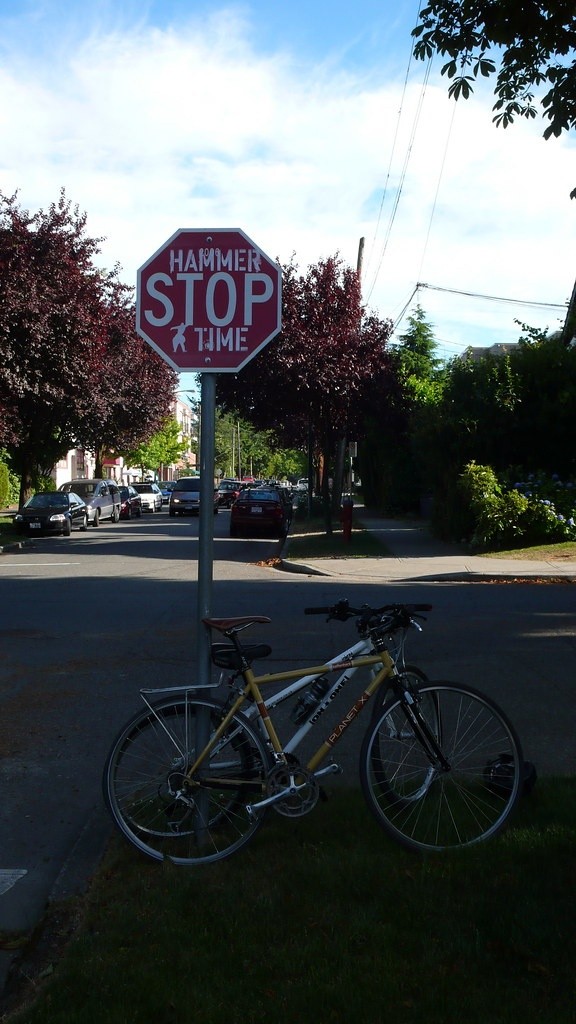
[13,492,88,536]
[253,477,308,516]
[119,486,142,521]
[131,481,163,513]
[157,481,175,504]
[218,480,252,507]
[229,489,286,538]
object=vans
[169,477,219,515]
[58,480,121,527]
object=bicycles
[103,599,524,869]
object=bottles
[287,676,330,727]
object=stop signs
[136,229,283,374]
